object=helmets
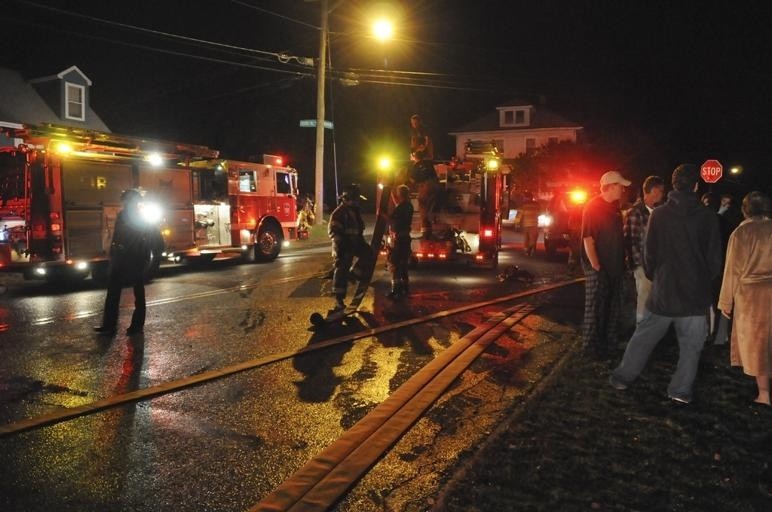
[119,189,142,202]
[337,186,367,201]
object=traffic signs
[324,120,333,130]
[299,119,316,127]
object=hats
[600,171,632,186]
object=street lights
[313,3,398,224]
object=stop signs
[699,160,723,182]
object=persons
[383,183,415,299]
[514,191,543,259]
[93,189,165,336]
[622,175,668,330]
[579,170,633,363]
[719,193,742,230]
[327,183,375,320]
[607,163,729,406]
[699,190,722,213]
[716,191,772,407]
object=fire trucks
[545,186,591,258]
[377,138,507,268]
[0,117,300,277]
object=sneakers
[670,392,689,407]
[347,265,364,280]
[127,322,143,332]
[94,324,114,332]
[608,376,627,390]
[386,284,409,298]
